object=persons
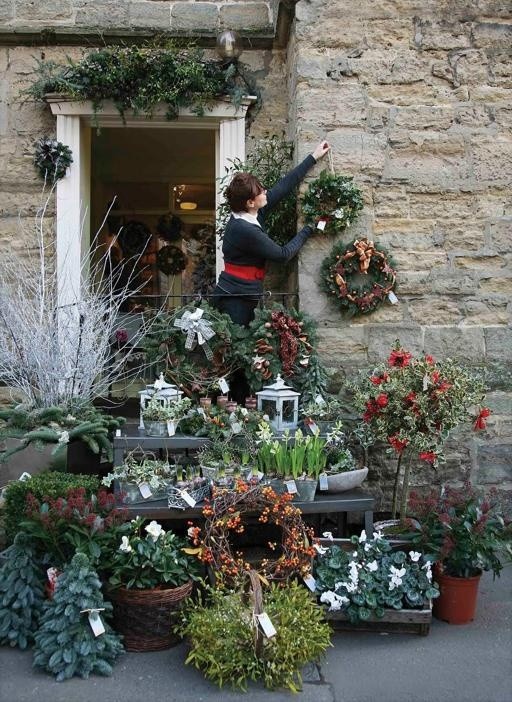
[206,140,332,406]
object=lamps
[180,201,197,211]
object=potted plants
[1,336,512,693]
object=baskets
[201,574,297,680]
[117,579,193,652]
